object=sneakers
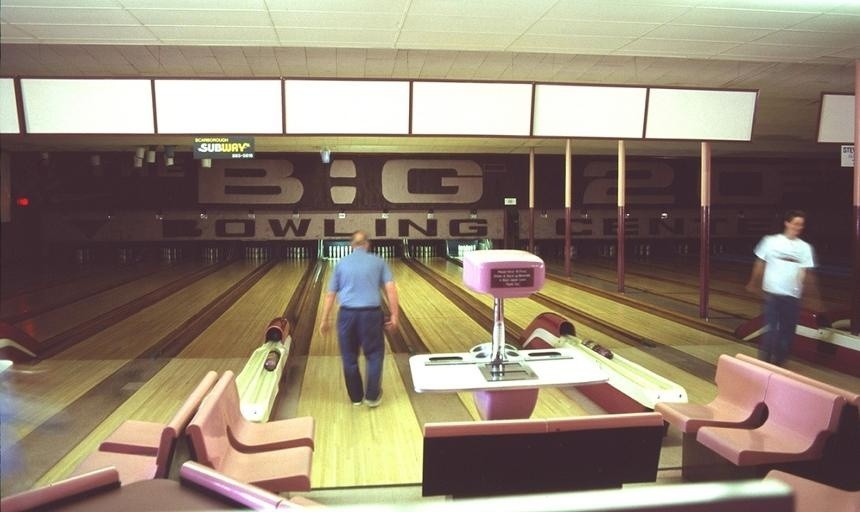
[367,388,384,407]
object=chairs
[56,369,317,494]
[654,352,860,491]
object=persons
[745,210,814,367]
[319,231,398,406]
